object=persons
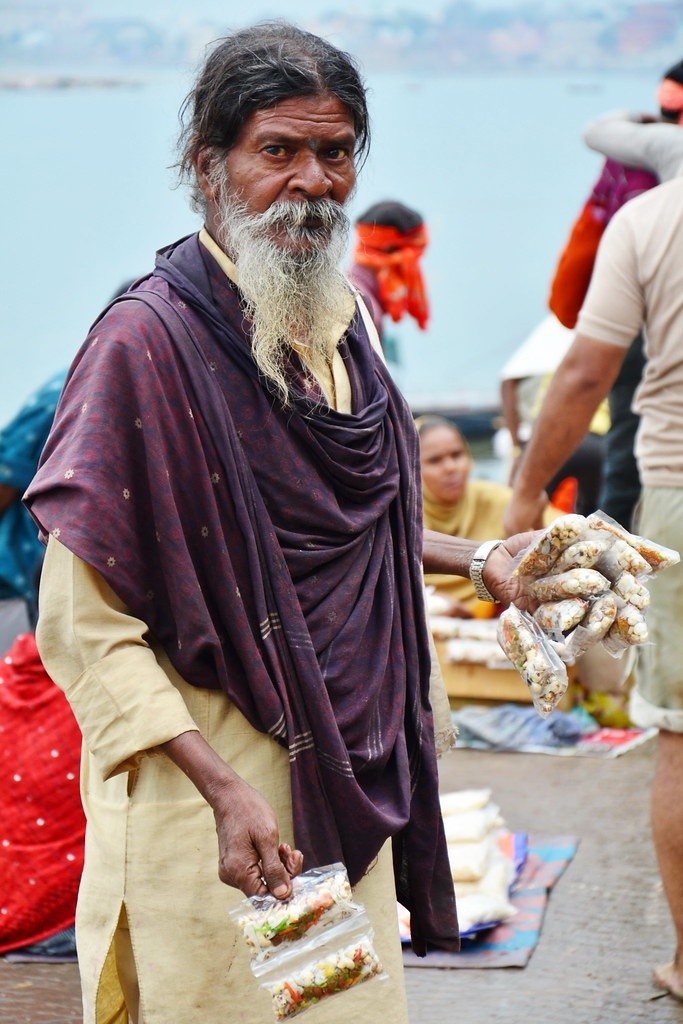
[501,177,683,1001]
[0,368,68,633]
[22,23,459,1024]
[413,415,567,618]
[583,61,683,531]
[348,202,430,335]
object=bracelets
[469,539,501,604]
